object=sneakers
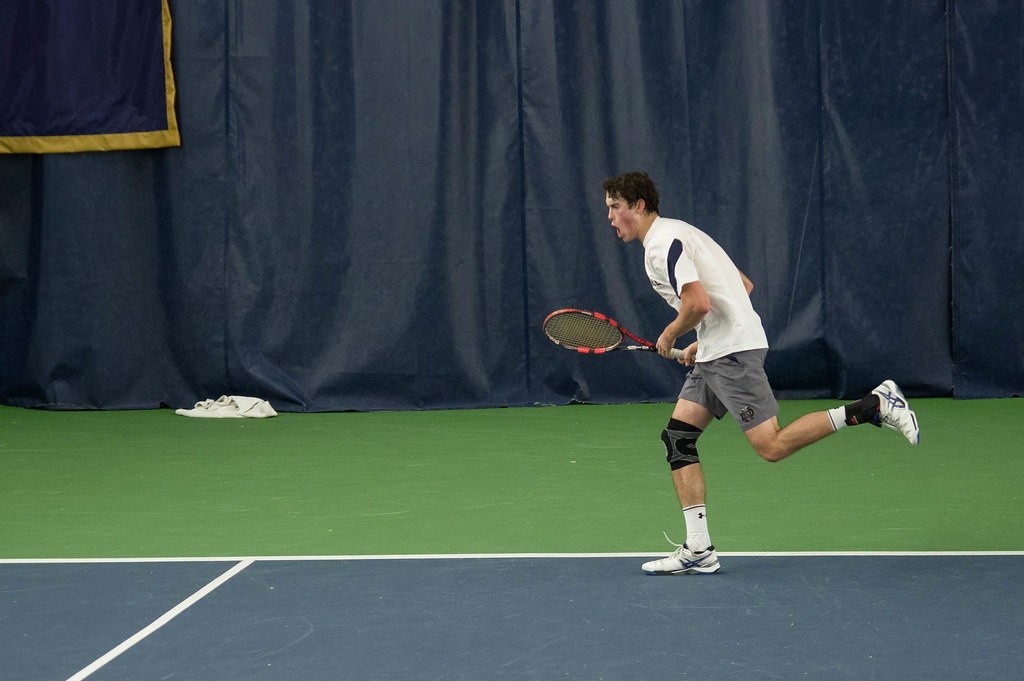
[641,544,721,575]
[870,380,920,447]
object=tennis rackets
[542,308,696,361]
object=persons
[601,172,921,574]
